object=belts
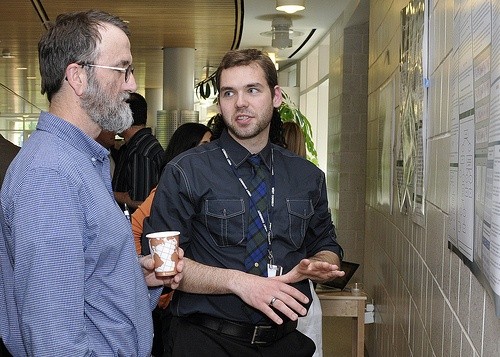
[186,311,298,346]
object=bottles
[124,211,130,222]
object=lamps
[275,0,306,13]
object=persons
[125,122,214,357]
[280,122,308,160]
[140,48,346,357]
[93,128,120,199]
[0,9,185,356]
[111,93,165,230]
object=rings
[269,297,278,306]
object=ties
[246,153,272,276]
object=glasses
[64,63,135,84]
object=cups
[350,283,361,296]
[146,231,180,276]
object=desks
[315,283,367,357]
